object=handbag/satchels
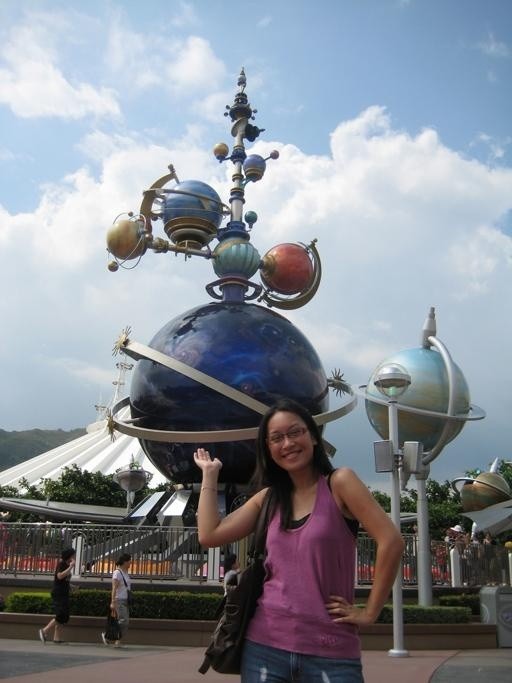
[197,565,266,675]
[104,616,122,641]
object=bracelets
[200,485,218,492]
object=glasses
[265,427,308,445]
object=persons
[221,553,241,594]
[102,553,133,649]
[191,395,406,682]
[40,547,77,644]
[402,523,511,585]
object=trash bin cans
[479,582,512,648]
